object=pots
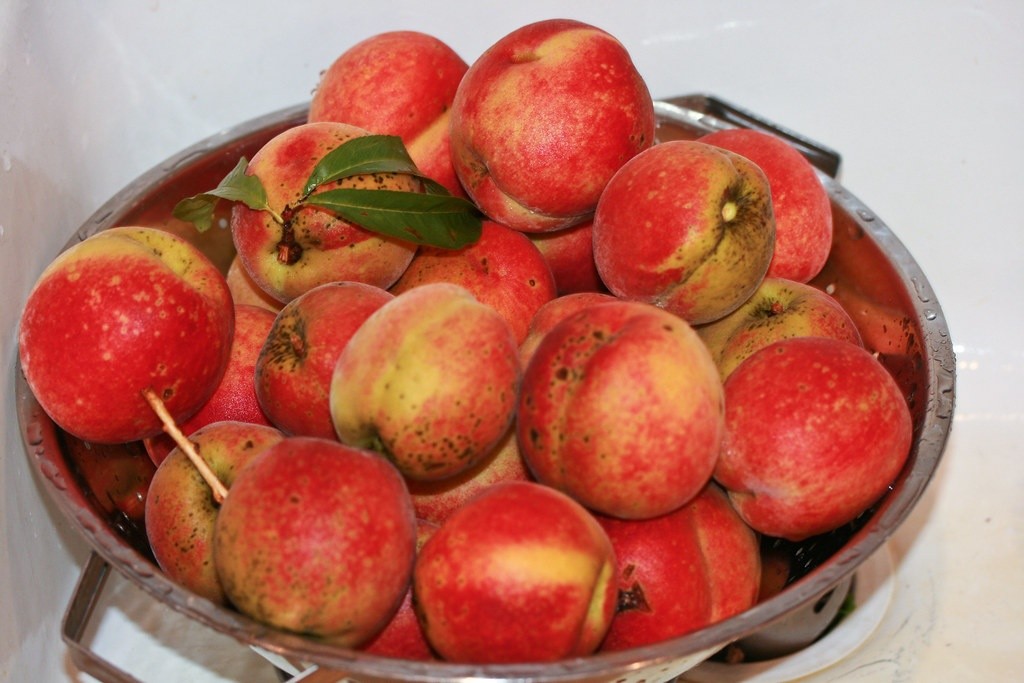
[11,87,946,682]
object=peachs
[19,18,915,664]
